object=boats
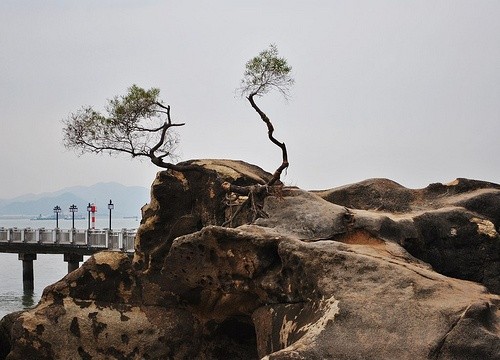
[63,215,85,220]
[32,213,57,220]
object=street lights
[68,204,78,229]
[53,205,62,229]
[87,203,92,229]
[107,199,113,230]
